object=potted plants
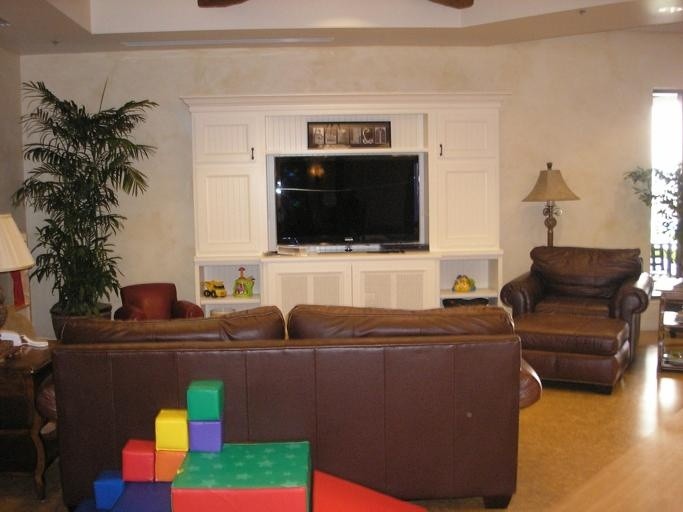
[4,75,160,340]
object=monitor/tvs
[267,151,425,253]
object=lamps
[521,160,580,246]
[1,212,35,361]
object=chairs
[498,245,654,396]
[112,281,204,320]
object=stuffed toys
[452,275,476,292]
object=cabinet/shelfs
[430,107,502,251]
[193,104,267,255]
[193,256,504,319]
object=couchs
[40,302,541,509]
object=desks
[0,338,52,500]
[655,287,683,375]
